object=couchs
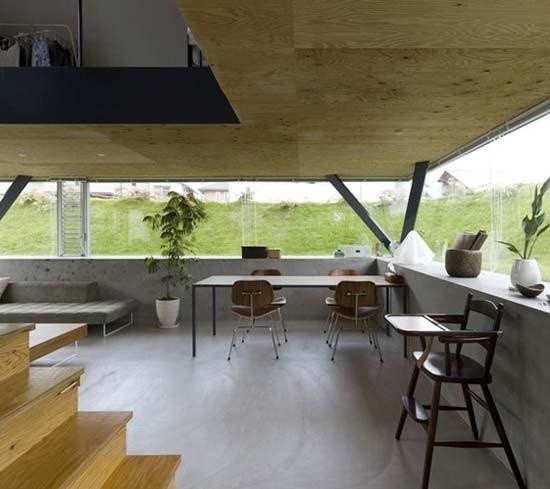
[0,281,137,337]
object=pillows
[0,277,10,300]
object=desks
[192,275,414,358]
[30,322,88,367]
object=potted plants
[495,177,550,288]
[142,190,211,327]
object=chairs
[384,294,525,489]
[227,269,382,361]
